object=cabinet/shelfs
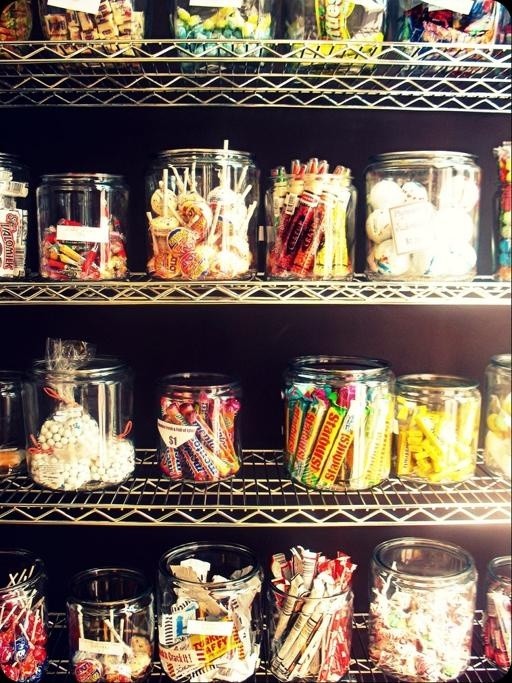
[0,36,511,681]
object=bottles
[391,371,479,484]
[1,144,33,282]
[365,149,482,282]
[159,539,263,681]
[146,147,261,282]
[1,550,47,680]
[0,0,511,71]
[487,143,512,279]
[37,169,130,284]
[279,354,392,492]
[157,372,245,484]
[368,537,478,683]
[262,160,358,280]
[266,550,354,683]
[481,356,512,481]
[0,370,32,476]
[31,359,135,493]
[63,569,154,682]
[482,556,512,673]
